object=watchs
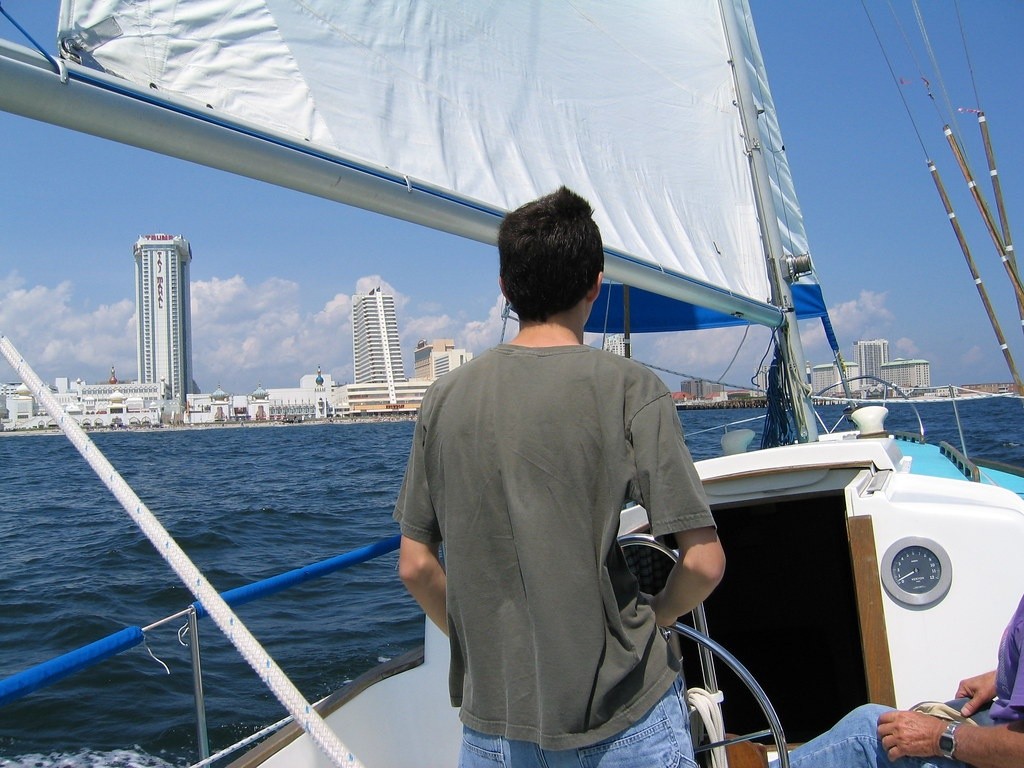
[939,720,962,760]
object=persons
[395,188,726,768]
[767,594,1024,768]
